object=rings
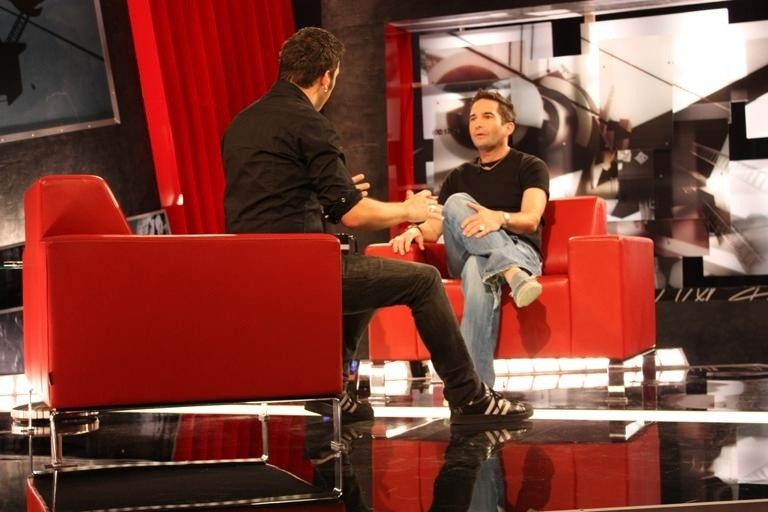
[429,203,437,213]
[394,236,400,240]
[479,224,485,231]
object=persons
[223,27,533,424]
[300,419,533,512]
[387,88,549,405]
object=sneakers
[450,381,533,425]
[509,271,542,308]
[305,382,373,420]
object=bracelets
[404,224,424,236]
[502,211,512,228]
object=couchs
[20,173,345,508]
[361,194,664,400]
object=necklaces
[480,147,511,171]
